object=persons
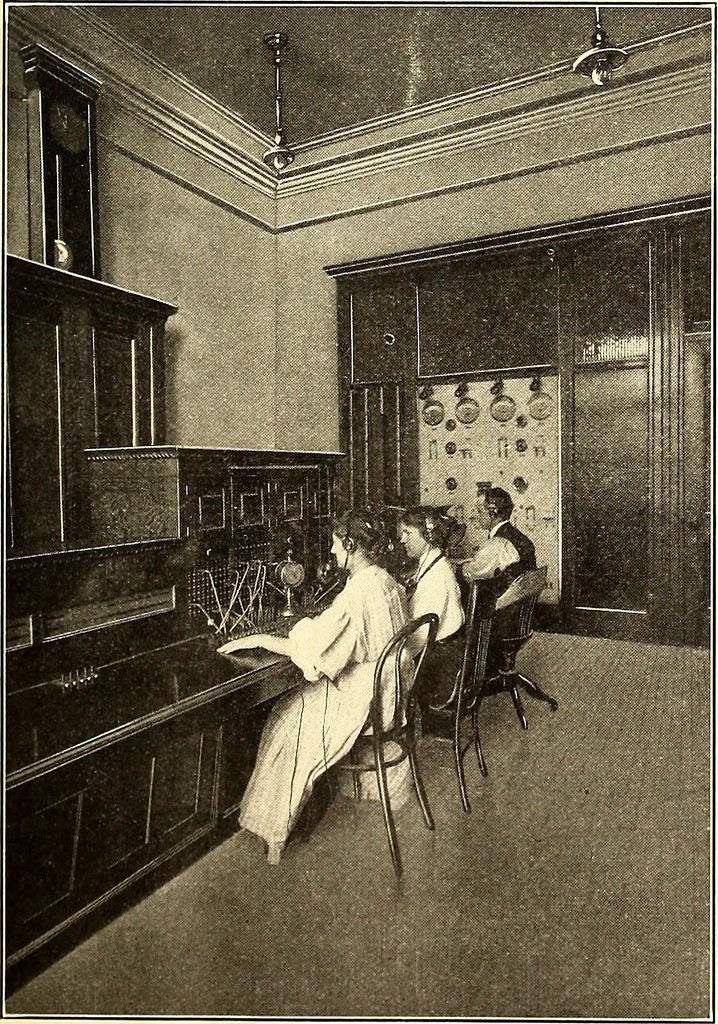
[441,485,535,688]
[213,507,416,856]
[380,508,469,726]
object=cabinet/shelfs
[88,447,346,633]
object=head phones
[484,490,503,519]
[423,507,437,545]
[341,507,367,553]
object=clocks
[51,99,90,156]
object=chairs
[301,613,439,875]
[494,566,557,729]
[421,578,502,812]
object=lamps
[263,33,296,173]
[575,7,630,86]
